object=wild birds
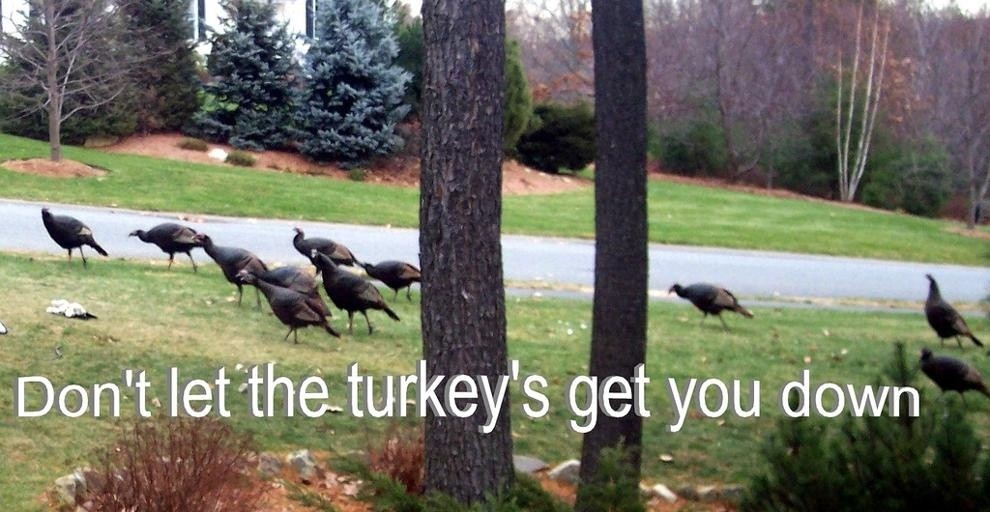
[919,347,989,409]
[926,274,986,351]
[669,285,753,334]
[42,209,107,270]
[129,224,197,273]
[192,228,421,343]
